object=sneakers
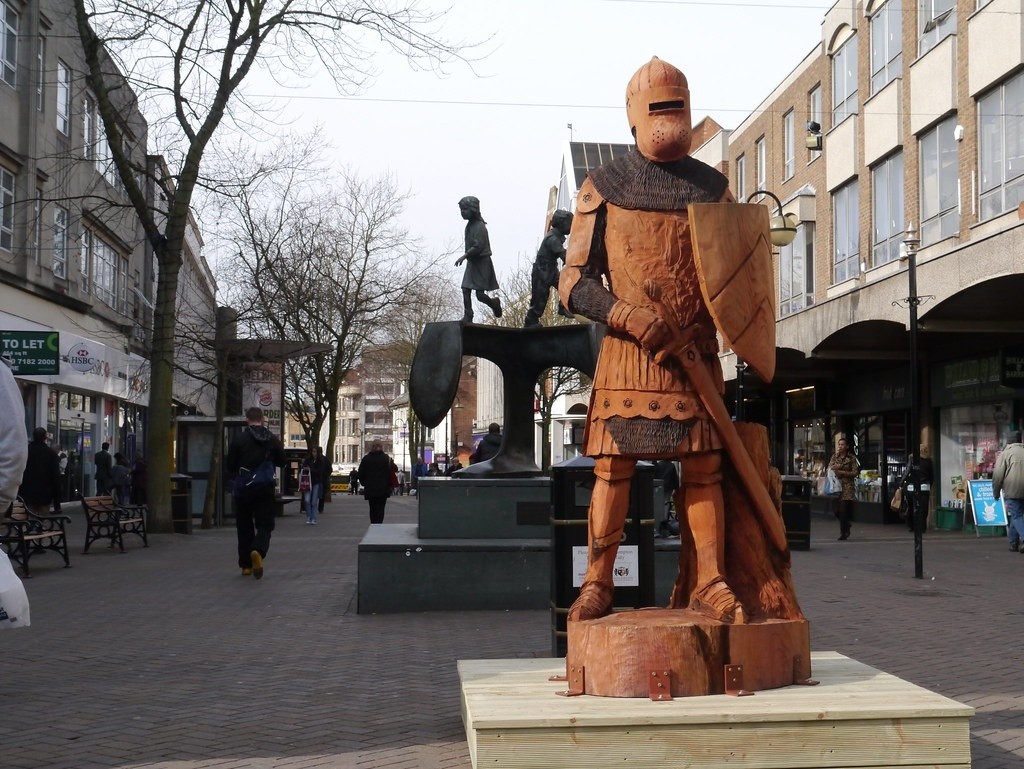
[242,568,251,575]
[250,551,263,580]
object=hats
[1006,431,1022,444]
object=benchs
[81,494,149,554]
[0,495,73,579]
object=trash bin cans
[170,474,192,535]
[780,474,813,551]
[551,454,656,659]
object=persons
[358,439,391,524]
[898,443,934,534]
[94,442,147,506]
[0,360,31,631]
[299,446,323,524]
[557,55,776,623]
[454,196,503,322]
[396,469,405,496]
[226,407,287,580]
[524,209,575,328]
[827,438,861,540]
[318,447,332,512]
[992,430,1024,554]
[470,423,503,466]
[349,467,357,495]
[413,457,463,500]
[18,427,61,554]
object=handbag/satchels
[890,487,902,512]
[817,477,825,496]
[388,457,399,488]
[824,468,842,495]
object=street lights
[352,428,362,459]
[391,418,405,474]
[735,191,798,423]
[445,396,464,469]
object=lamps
[805,120,821,135]
[806,136,823,151]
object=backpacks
[236,445,276,494]
[297,467,312,491]
